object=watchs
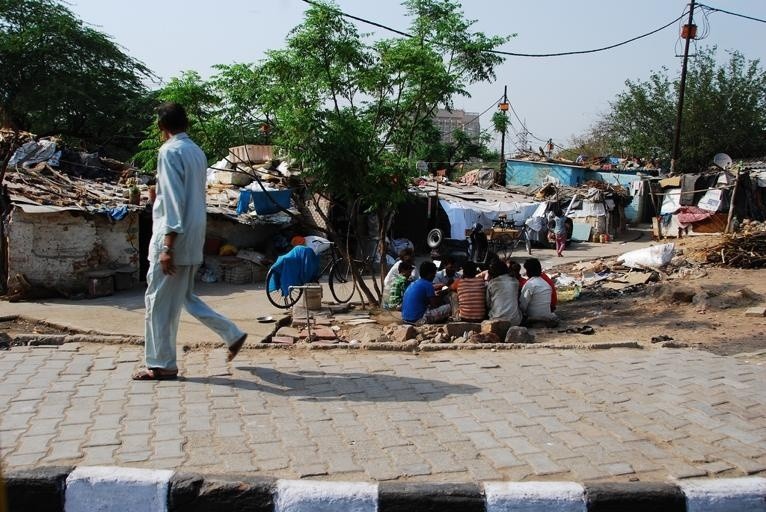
[160,246,172,253]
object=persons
[381,246,559,328]
[554,207,571,256]
[130,103,249,379]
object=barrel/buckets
[303,283,321,308]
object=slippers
[132,365,177,380]
[227,330,248,363]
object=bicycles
[490,210,521,253]
[493,222,532,261]
[265,240,356,311]
